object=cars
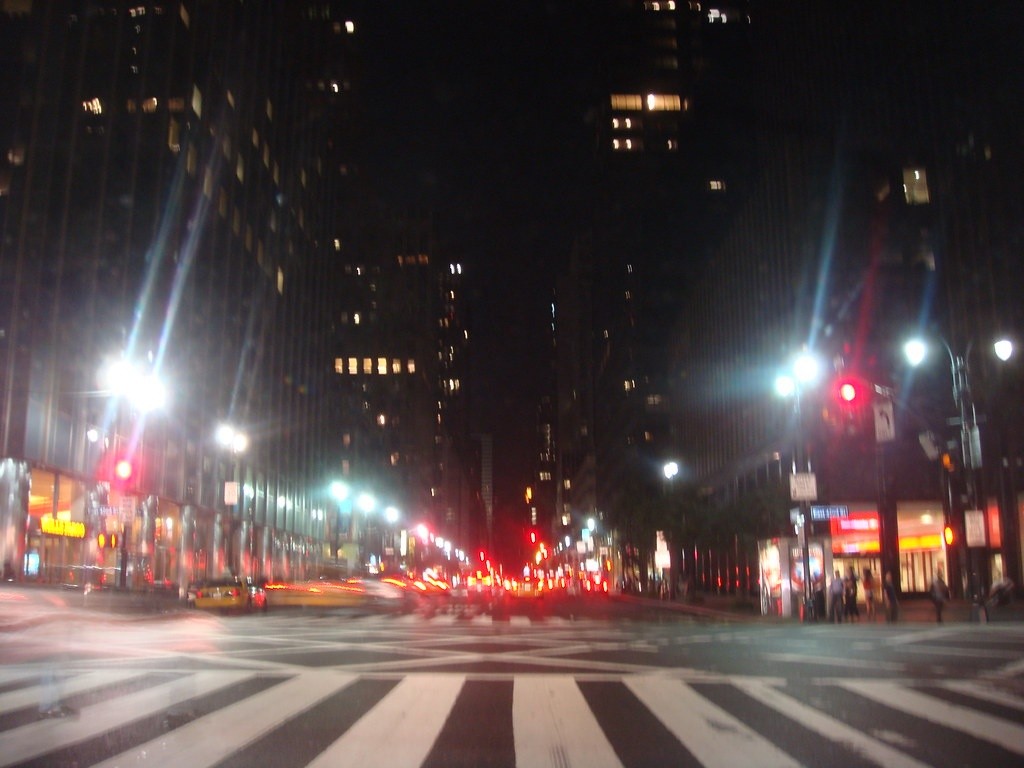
[195,579,268,613]
[274,577,445,621]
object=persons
[812,570,824,624]
[964,570,990,621]
[928,569,950,622]
[882,570,901,625]
[827,570,843,623]
[862,567,877,620]
[843,568,860,623]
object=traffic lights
[834,374,876,414]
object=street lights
[661,460,679,601]
[98,345,170,593]
[903,330,1011,623]
[214,426,250,578]
[776,348,821,625]
[324,472,466,568]
